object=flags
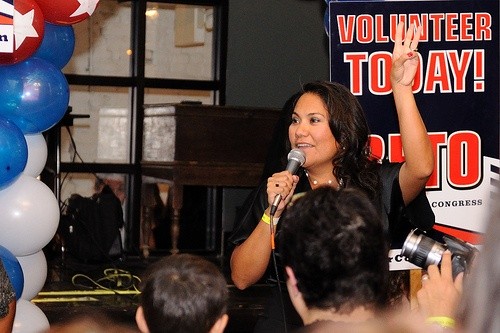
[0,0,14,53]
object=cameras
[401,228,479,282]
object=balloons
[24,133,48,179]
[0,177,60,257]
[36,0,99,26]
[0,245,24,302]
[0,56,70,135]
[0,0,45,65]
[15,251,48,300]
[30,22,75,70]
[0,114,28,190]
[11,300,50,333]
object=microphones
[270,148,306,216]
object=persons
[228,21,434,333]
[134,254,231,333]
[417,173,500,333]
[0,260,16,333]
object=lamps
[146,2,160,20]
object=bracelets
[261,213,279,225]
[425,315,454,328]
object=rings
[421,276,427,280]
[410,47,418,52]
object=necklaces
[304,169,336,185]
